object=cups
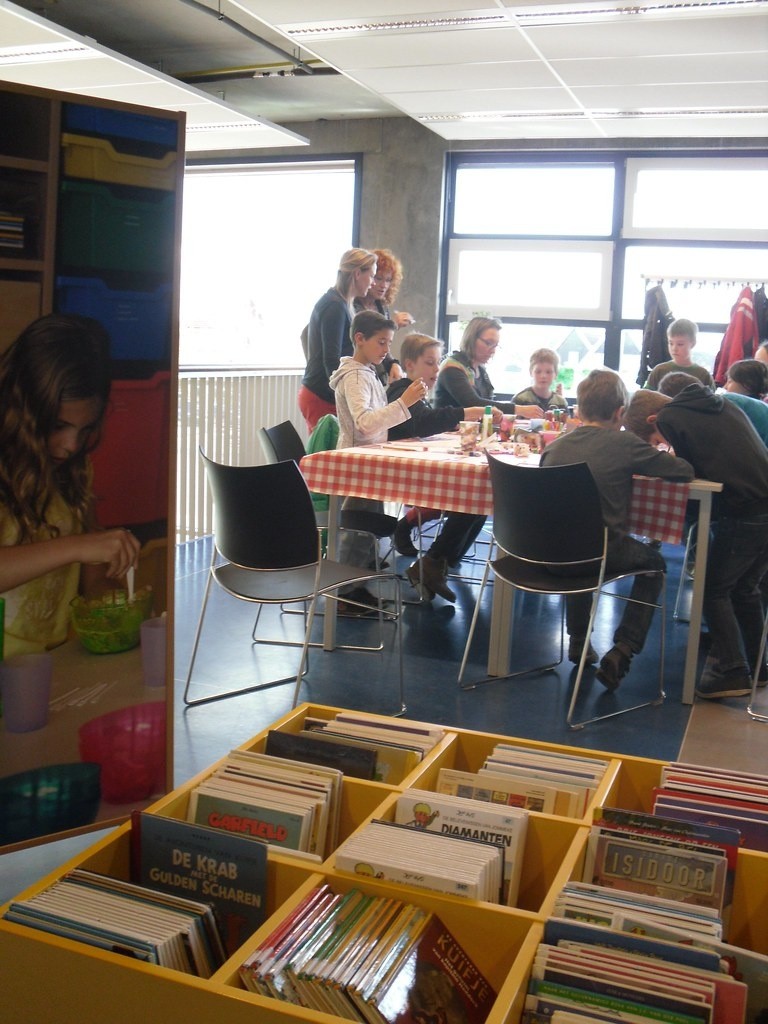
[140,617,167,686]
[459,420,480,454]
[1,652,53,733]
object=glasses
[477,336,497,350]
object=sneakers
[335,588,388,616]
[406,556,456,602]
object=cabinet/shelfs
[0,80,187,359]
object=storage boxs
[51,102,178,531]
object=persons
[298,248,768,699]
[0,310,142,676]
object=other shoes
[751,664,768,689]
[595,647,632,691]
[569,635,599,665]
[389,516,420,557]
[693,666,752,698]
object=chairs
[458,448,666,730]
[183,414,495,719]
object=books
[2,711,768,1024]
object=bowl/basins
[1,760,101,846]
[79,700,166,805]
[69,584,154,655]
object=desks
[0,636,172,827]
[299,429,723,706]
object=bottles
[482,405,493,439]
